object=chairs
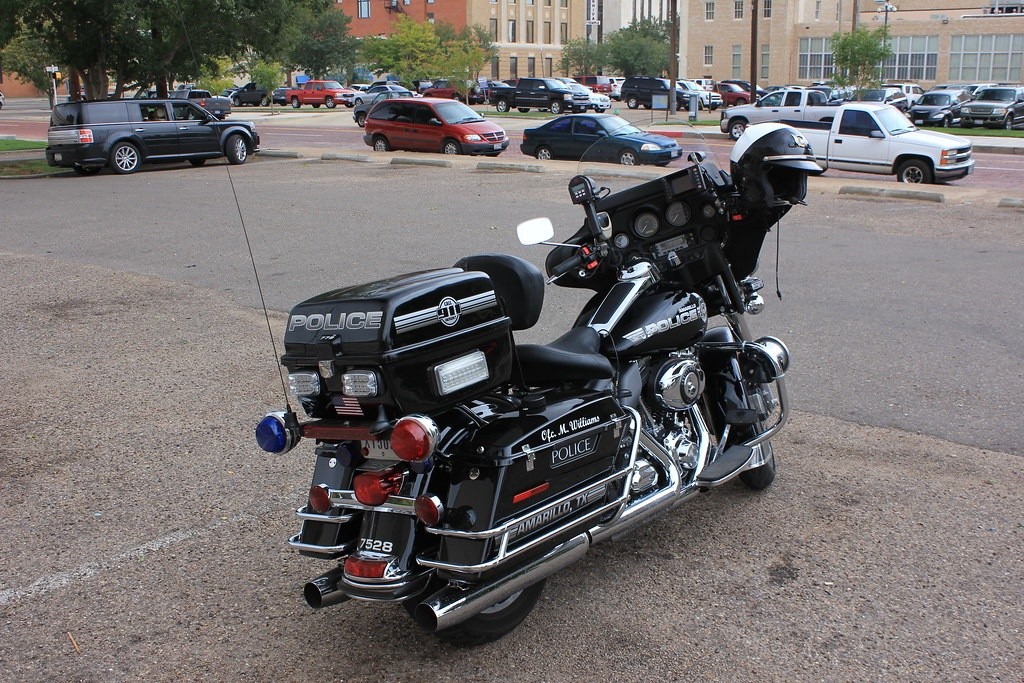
[140,108,166,120]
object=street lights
[877,0,897,81]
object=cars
[765,81,924,112]
[178,79,497,127]
[46,98,260,174]
[711,83,761,106]
[904,91,970,128]
[961,86,1024,131]
[538,77,612,113]
[927,82,999,96]
[721,80,769,98]
[677,81,723,110]
[609,77,625,101]
[572,76,612,96]
[520,112,682,167]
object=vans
[363,98,510,157]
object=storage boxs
[279,269,513,422]
[299,443,368,561]
[435,390,632,585]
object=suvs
[620,75,699,111]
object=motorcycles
[255,118,792,643]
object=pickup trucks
[745,102,976,184]
[169,89,231,120]
[487,78,591,114]
